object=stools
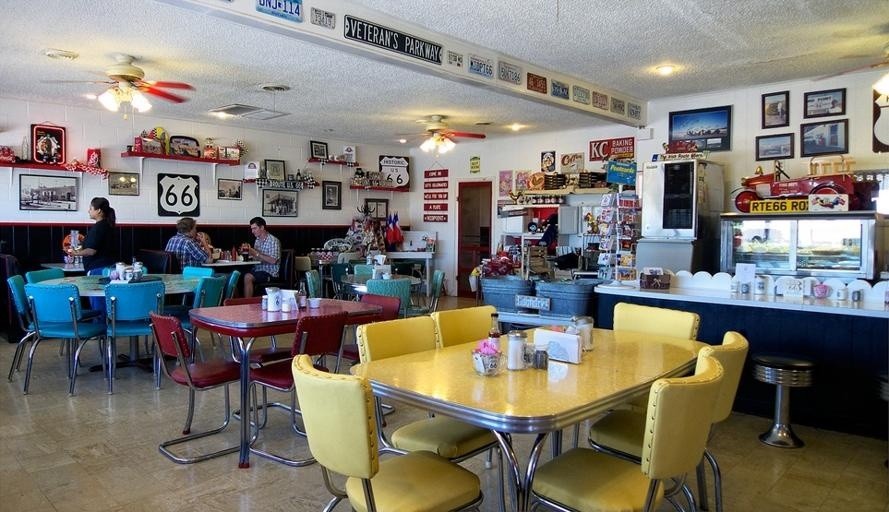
[754,355,817,450]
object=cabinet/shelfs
[635,238,720,279]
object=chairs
[223,296,302,430]
[400,269,445,317]
[322,253,361,299]
[162,266,215,365]
[136,249,177,275]
[432,306,501,468]
[165,276,225,367]
[354,264,374,275]
[252,249,296,296]
[306,269,321,298]
[25,268,105,367]
[8,274,80,381]
[295,257,311,271]
[24,283,108,398]
[292,353,485,512]
[328,293,400,416]
[613,301,700,341]
[102,281,165,395]
[208,270,240,361]
[149,310,259,464]
[357,315,507,512]
[102,265,149,355]
[366,279,412,319]
[248,310,348,467]
[589,331,748,512]
[533,355,724,512]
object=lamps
[871,74,889,95]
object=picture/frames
[761,91,789,129]
[322,180,342,210]
[803,88,846,119]
[30,124,66,166]
[310,140,328,159]
[19,174,78,211]
[364,198,389,220]
[170,135,201,159]
[669,104,731,152]
[263,159,286,181]
[262,189,299,217]
[217,178,242,200]
[800,119,848,157]
[108,172,140,196]
[755,133,794,161]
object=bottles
[75,255,84,265]
[321,248,326,256]
[134,263,144,276]
[315,248,321,255]
[884,281,889,307]
[508,331,528,370]
[116,263,125,272]
[220,250,232,260]
[125,269,133,280]
[232,247,237,261]
[297,282,307,312]
[262,296,268,310]
[366,252,373,265]
[488,313,501,351]
[326,248,332,256]
[242,244,250,262]
[282,297,292,312]
[576,316,593,350]
[754,275,767,296]
[268,287,281,312]
[21,136,28,161]
[310,248,316,255]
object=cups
[307,297,322,309]
[754,277,768,295]
[812,284,830,298]
[67,229,79,248]
[739,280,752,295]
[852,291,862,302]
[729,280,739,293]
[836,288,849,301]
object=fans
[393,114,486,154]
[39,55,196,114]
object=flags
[394,212,404,251]
[386,212,396,252]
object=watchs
[255,252,258,257]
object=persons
[165,218,238,298]
[241,217,280,298]
[195,231,210,247]
[68,197,116,325]
